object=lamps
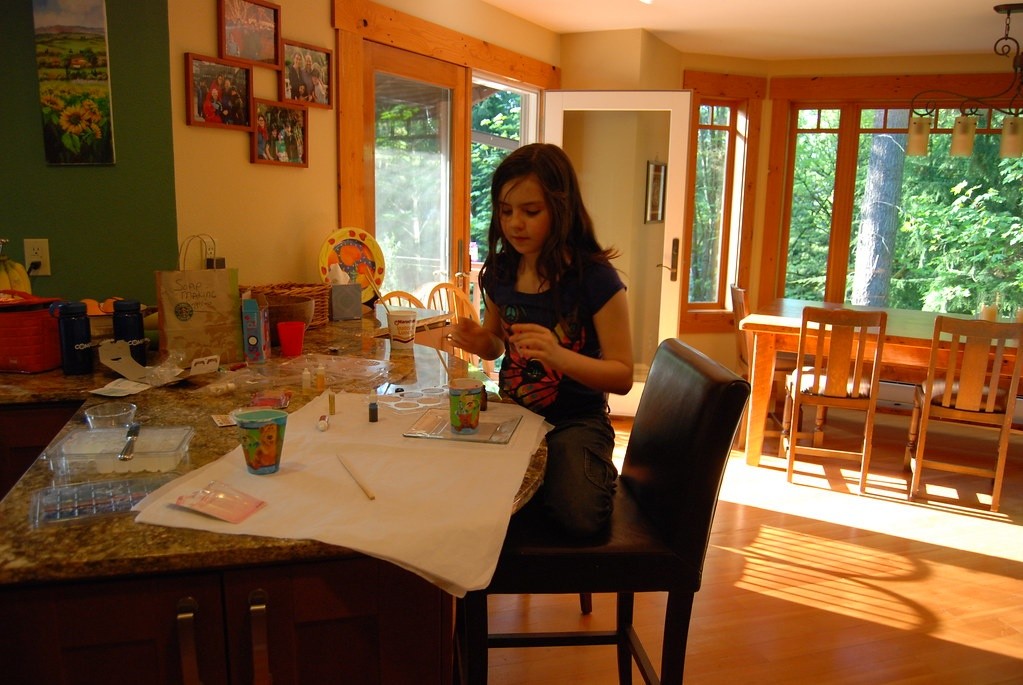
[906,3,1022,157]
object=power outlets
[23,237,51,276]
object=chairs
[730,284,803,452]
[903,316,1023,512]
[455,338,751,685]
[778,306,888,492]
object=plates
[402,408,524,445]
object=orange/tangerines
[80,297,122,315]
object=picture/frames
[277,38,334,110]
[216,0,282,71]
[250,97,308,168]
[184,52,254,132]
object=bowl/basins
[142,306,159,349]
[266,295,315,347]
[85,402,137,429]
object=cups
[232,409,289,475]
[386,310,417,350]
[448,378,483,435]
[278,321,305,358]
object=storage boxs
[0,290,65,373]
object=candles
[1015,309,1023,321]
[979,307,997,322]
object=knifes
[118,420,140,461]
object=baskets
[239,282,332,330]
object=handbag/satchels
[157,233,245,369]
[0,289,64,373]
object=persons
[451,142,633,542]
[258,114,299,162]
[196,75,245,124]
[228,19,261,57]
[289,52,325,102]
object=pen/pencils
[337,454,374,500]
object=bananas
[0,256,33,298]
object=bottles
[49,300,95,377]
[98,297,147,367]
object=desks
[0,325,548,685]
[739,297,1023,467]
